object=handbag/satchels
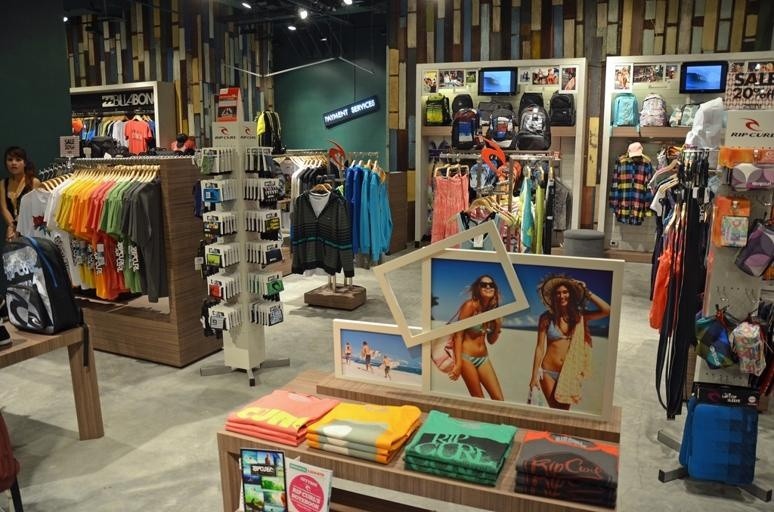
[430,322,463,375]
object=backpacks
[424,89,578,152]
[610,90,705,130]
[2,234,90,368]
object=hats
[627,142,644,158]
[541,274,587,312]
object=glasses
[478,281,498,290]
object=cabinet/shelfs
[199,147,284,330]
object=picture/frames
[422,247,625,423]
[613,62,633,94]
[422,65,579,95]
[333,318,423,392]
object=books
[284,456,334,512]
[239,447,287,512]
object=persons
[528,282,611,411]
[362,342,372,370]
[520,67,557,87]
[0,147,43,244]
[423,71,457,95]
[383,356,391,379]
[448,275,504,403]
[615,64,657,90]
[344,341,352,365]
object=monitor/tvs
[478,67,518,96]
[679,60,728,94]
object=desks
[0,318,106,441]
[217,370,622,512]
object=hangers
[285,150,384,192]
[37,115,161,192]
[434,153,555,179]
[629,146,678,171]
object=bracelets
[582,287,592,299]
